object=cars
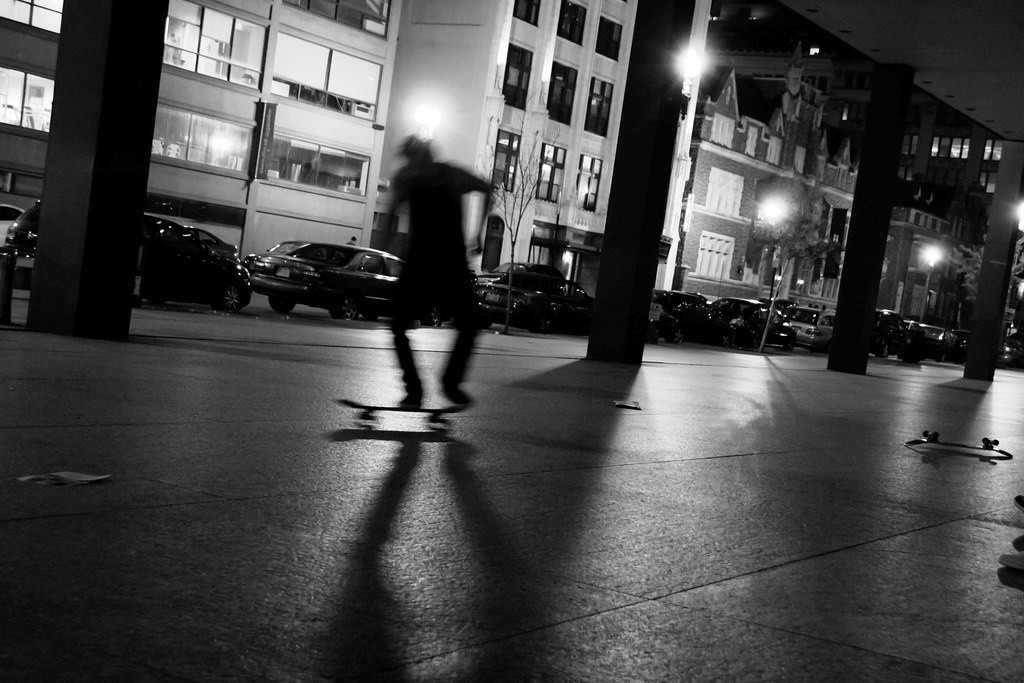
[5,198,253,313]
[0,203,26,248]
[646,287,1024,368]
[242,239,445,326]
[182,224,241,265]
[476,259,595,335]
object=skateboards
[904,430,1014,459]
[334,394,484,429]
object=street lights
[762,195,789,320]
[919,244,942,325]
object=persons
[346,236,357,245]
[380,134,495,409]
